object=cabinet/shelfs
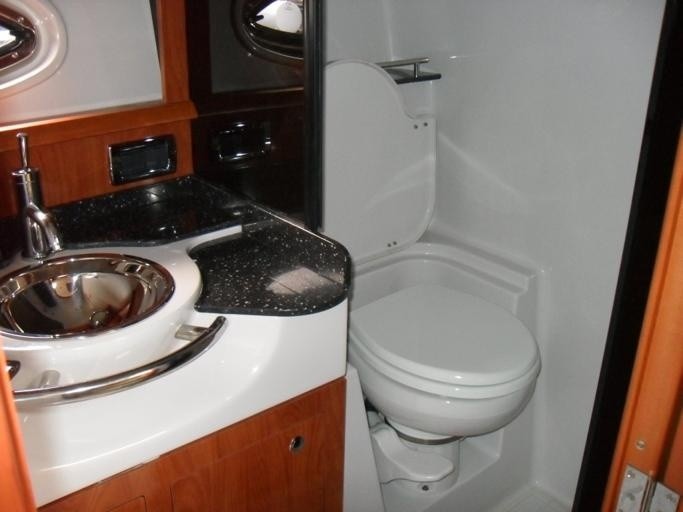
[37,377,345,512]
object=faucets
[10,131,67,258]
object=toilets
[322,57,540,494]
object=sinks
[0,1,68,99]
[0,250,176,343]
